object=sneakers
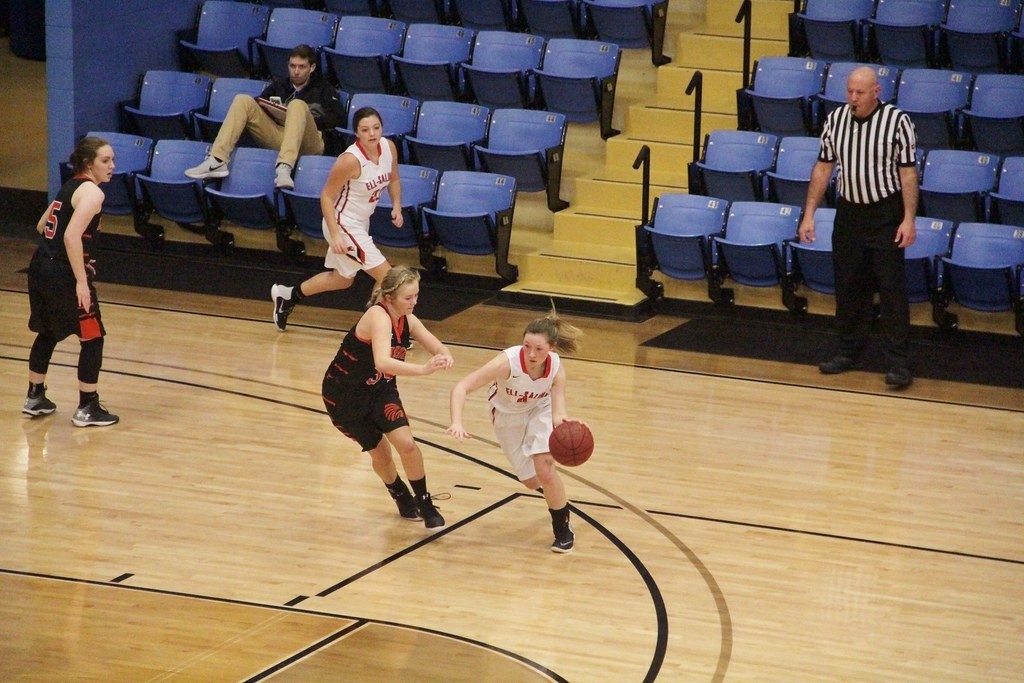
[274,163,294,190]
[551,510,575,551]
[271,283,301,332]
[22,418,53,448]
[413,492,451,529]
[388,482,424,521]
[22,385,56,416]
[885,366,913,385]
[71,393,119,427]
[391,333,413,350]
[185,155,229,179]
[818,355,855,374]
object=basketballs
[548,421,595,467]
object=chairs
[59,0,1024,335]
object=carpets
[13,248,502,322]
[637,319,1024,389]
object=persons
[799,67,918,384]
[184,44,343,188]
[445,312,592,553]
[271,107,413,349]
[22,137,120,427]
[322,266,454,531]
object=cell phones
[270,96,282,106]
